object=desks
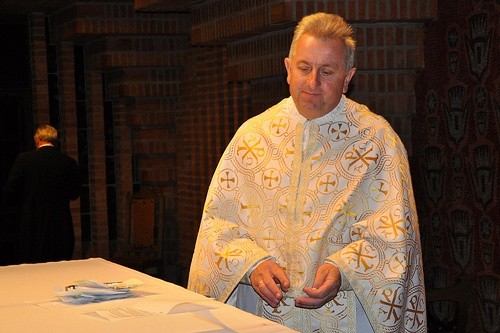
[0,257,301,333]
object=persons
[9,123,82,264]
[187,12,430,333]
